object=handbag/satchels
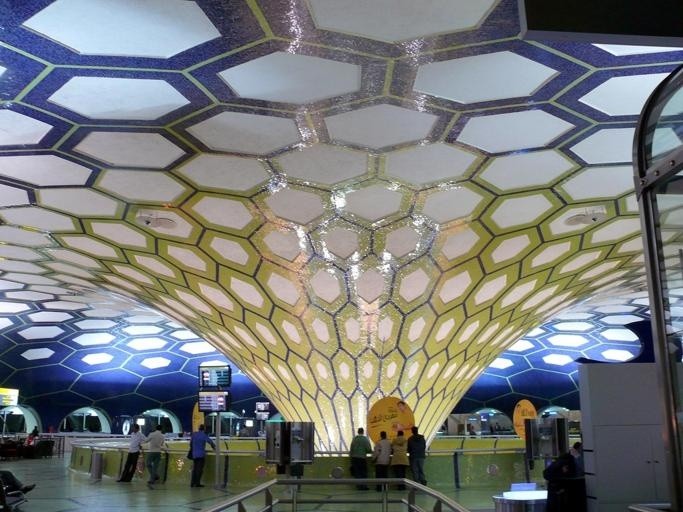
[187,445,194,460]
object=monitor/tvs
[255,412,270,422]
[245,419,253,427]
[256,402,271,411]
[198,391,230,412]
[198,366,232,387]
[135,418,145,425]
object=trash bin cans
[91,453,103,479]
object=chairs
[0,438,55,461]
[0,474,28,512]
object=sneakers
[22,484,36,494]
[191,484,206,488]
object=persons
[349,427,373,491]
[0,470,36,494]
[114,423,147,483]
[390,431,409,492]
[395,400,412,433]
[407,426,427,490]
[32,426,39,437]
[27,433,33,445]
[371,431,392,493]
[187,425,215,487]
[142,425,165,485]
